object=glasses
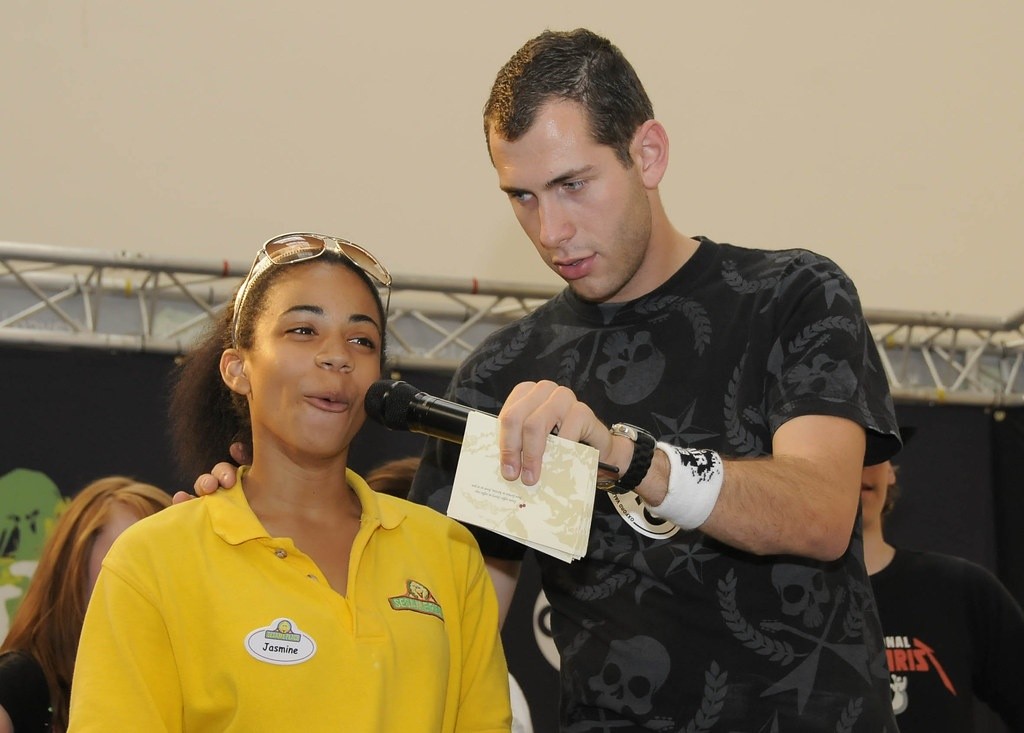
[230,231,392,351]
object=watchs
[607,422,657,495]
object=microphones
[362,379,620,474]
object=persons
[367,458,420,501]
[500,554,561,733]
[64,233,510,733]
[173,28,905,733]
[0,477,171,733]
[858,459,1024,733]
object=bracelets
[643,441,725,530]
